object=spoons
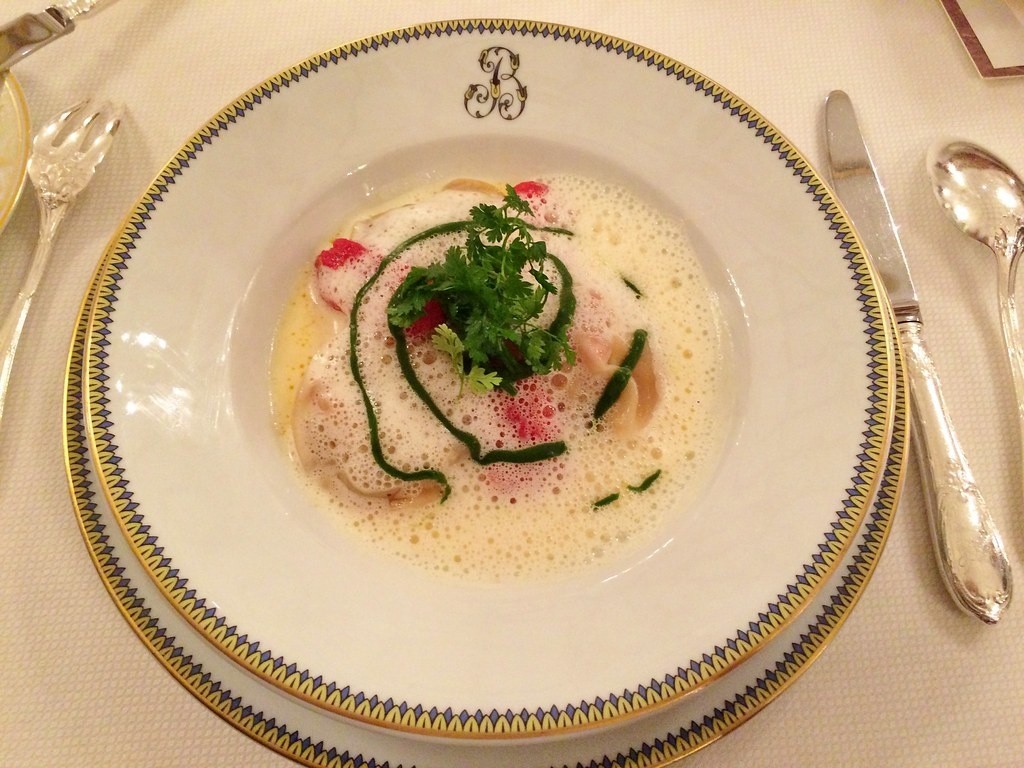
[923,140,1024,413]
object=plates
[63,241,910,768]
[91,16,895,743]
[0,70,32,235]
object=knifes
[0,0,119,71]
[825,88,1014,625]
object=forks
[0,100,120,420]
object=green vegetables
[384,183,577,399]
